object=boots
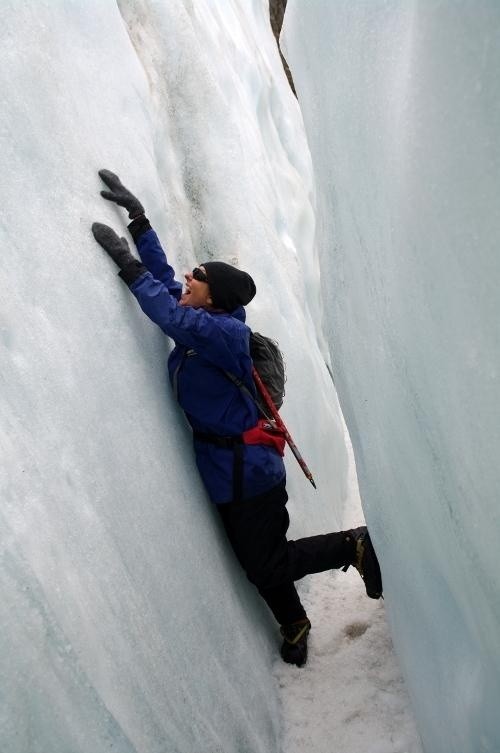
[347,526,383,600]
[280,618,311,667]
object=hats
[200,261,255,309]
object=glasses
[192,267,208,283]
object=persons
[91,170,383,668]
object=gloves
[98,169,145,219]
[92,222,136,270]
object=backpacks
[250,331,286,418]
